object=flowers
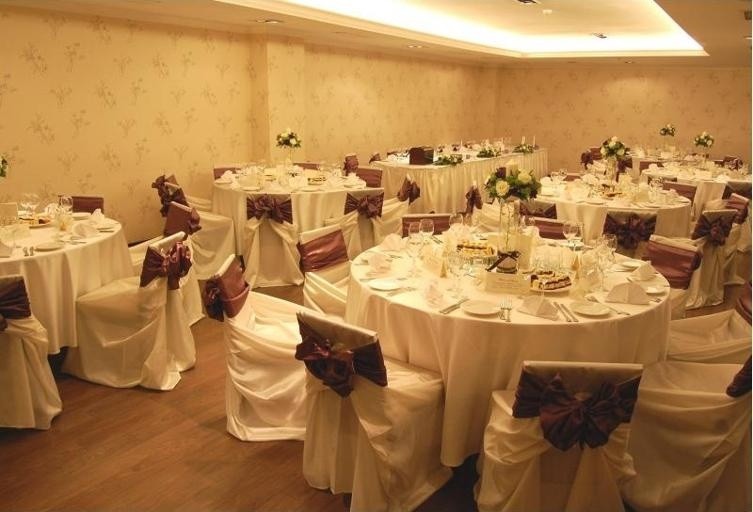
[658,122,676,138]
[690,128,718,148]
[273,126,304,147]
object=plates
[370,277,399,292]
[464,299,500,316]
[621,258,644,268]
[570,300,612,316]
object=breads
[531,268,571,290]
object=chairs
[0,195,204,432]
[149,138,751,508]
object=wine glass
[546,146,749,211]
[213,160,363,193]
[0,189,113,258]
[403,211,618,300]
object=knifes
[559,304,578,321]
[553,302,570,323]
